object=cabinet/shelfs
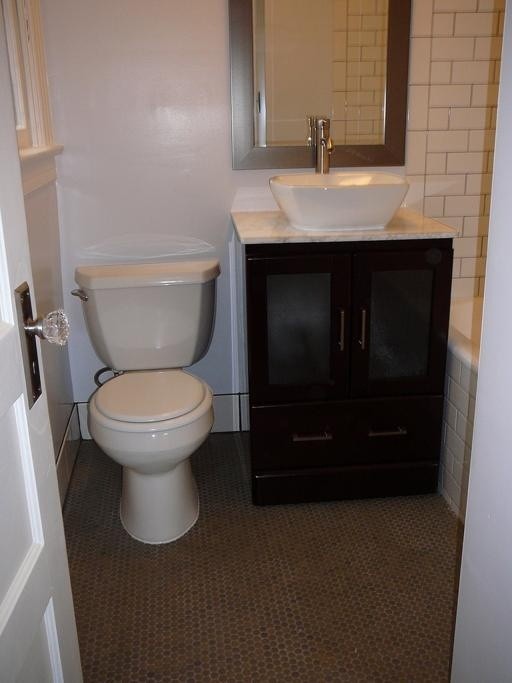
[233,244,455,508]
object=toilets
[70,259,239,547]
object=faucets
[315,118,333,174]
[308,116,321,146]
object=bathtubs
[446,297,483,370]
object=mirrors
[227,0,412,171]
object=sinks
[268,173,409,230]
